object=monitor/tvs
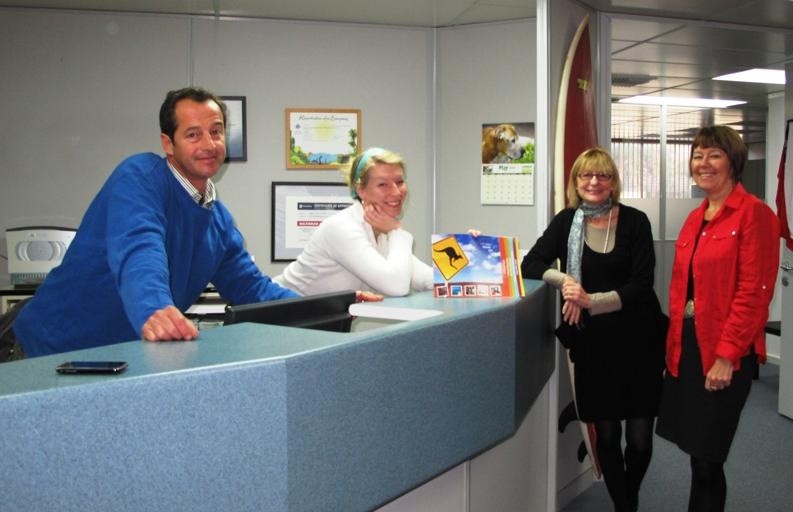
[224,289,357,332]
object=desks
[752,320,781,380]
[1,294,220,315]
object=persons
[9,85,384,361]
[519,147,670,512]
[652,123,784,511]
[270,148,482,299]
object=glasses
[578,171,615,182]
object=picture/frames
[270,180,351,263]
[216,94,247,163]
[283,107,363,170]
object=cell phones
[57,360,130,373]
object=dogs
[482,124,526,165]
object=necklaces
[603,209,612,253]
